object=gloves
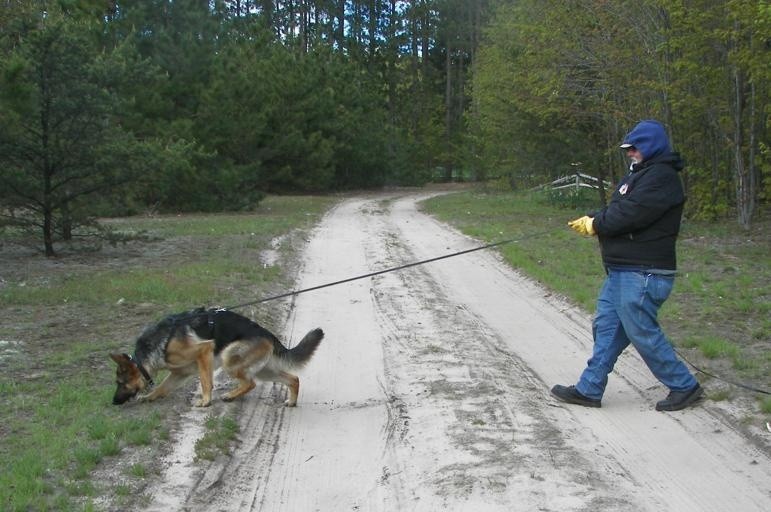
[568,215,596,238]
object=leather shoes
[551,384,602,408]
[655,381,704,411]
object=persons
[549,119,704,411]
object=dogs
[109,307,323,407]
